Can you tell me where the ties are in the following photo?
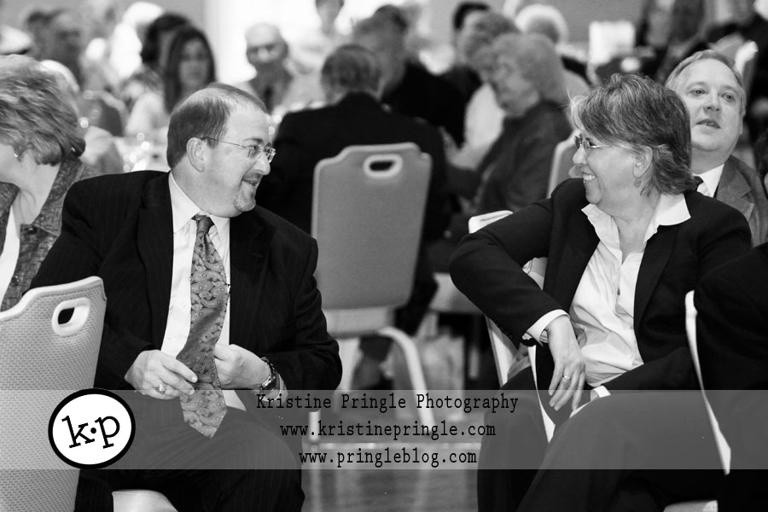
[692,176,703,190]
[176,213,228,439]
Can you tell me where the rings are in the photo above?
[563,373,572,380]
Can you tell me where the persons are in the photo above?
[1,54,103,312]
[127,17,187,113]
[662,48,767,246]
[352,33,572,389]
[440,0,574,118]
[665,2,766,59]
[310,0,410,43]
[247,25,298,110]
[259,46,447,388]
[355,20,442,122]
[453,76,768,512]
[129,24,217,142]
[0,1,84,77]
[29,85,343,511]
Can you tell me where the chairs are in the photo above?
[544,130,575,200]
[0,275,106,512]
[311,142,437,441]
[468,209,547,387]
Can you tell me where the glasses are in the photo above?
[573,133,616,153]
[201,136,277,163]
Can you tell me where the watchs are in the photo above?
[256,353,280,400]
